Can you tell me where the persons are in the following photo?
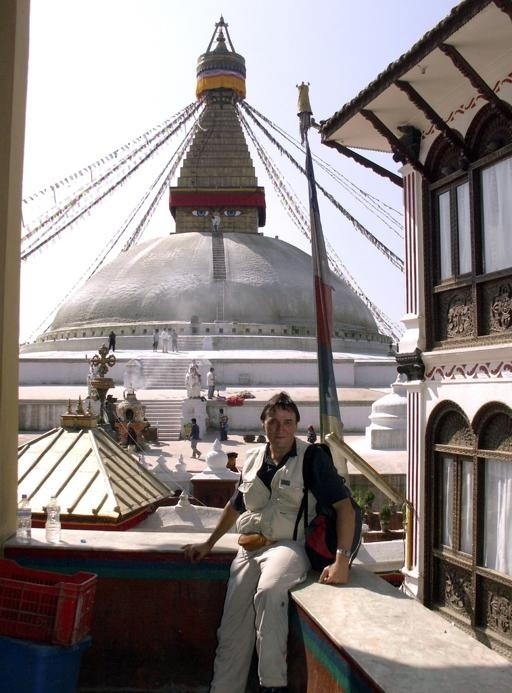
[153,327,161,352]
[108,330,116,351]
[219,407,228,440]
[189,418,201,459]
[161,327,169,352]
[180,391,357,693]
[306,424,316,443]
[207,366,216,398]
[171,329,178,351]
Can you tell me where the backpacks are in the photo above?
[293,442,363,571]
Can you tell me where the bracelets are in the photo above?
[205,539,213,548]
[336,547,350,557]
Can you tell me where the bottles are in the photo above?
[15,494,33,544]
[44,496,62,543]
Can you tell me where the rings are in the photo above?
[184,549,189,552]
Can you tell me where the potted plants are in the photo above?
[351,489,406,532]
